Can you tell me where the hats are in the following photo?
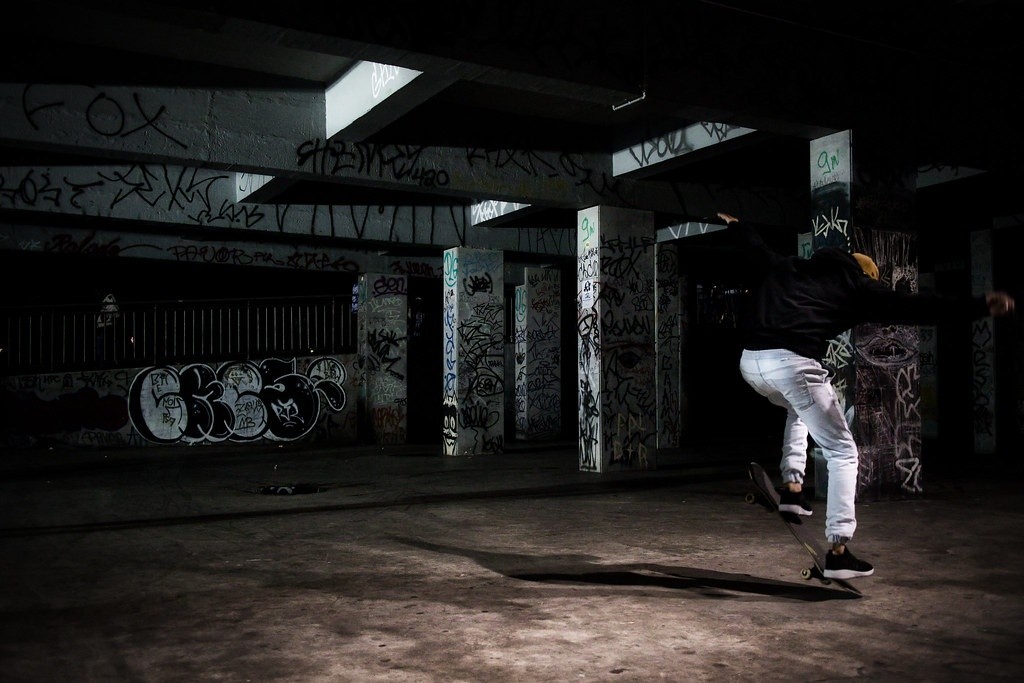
[851,252,880,281]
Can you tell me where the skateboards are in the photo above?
[744,461,864,598]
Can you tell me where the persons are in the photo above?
[717,211,1015,580]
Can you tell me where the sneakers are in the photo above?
[779,487,814,516]
[822,545,875,579]
